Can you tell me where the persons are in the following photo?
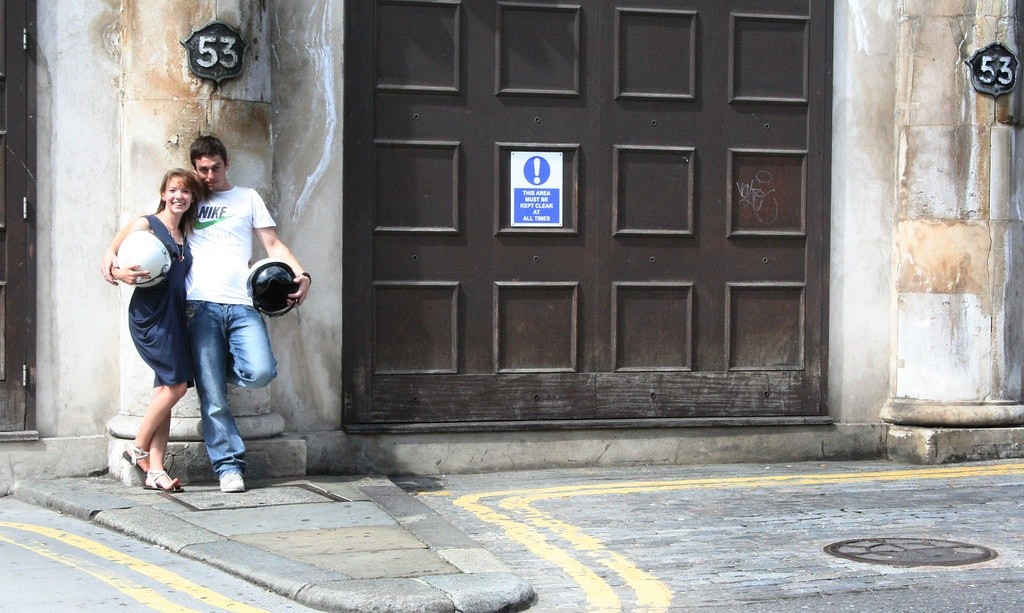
[102,132,313,495]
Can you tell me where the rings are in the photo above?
[136,276,142,282]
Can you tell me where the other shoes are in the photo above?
[221,472,244,492]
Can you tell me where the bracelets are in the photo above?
[300,272,312,284]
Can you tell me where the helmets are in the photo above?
[244,257,297,316]
[116,230,171,288]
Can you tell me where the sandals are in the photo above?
[145,472,185,492]
[123,441,149,475]
